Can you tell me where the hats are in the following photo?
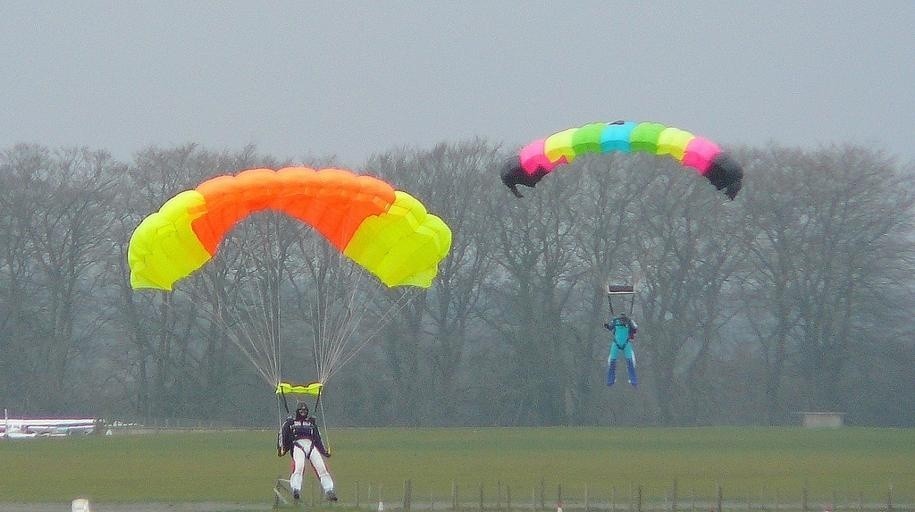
[296,401,309,412]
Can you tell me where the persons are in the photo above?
[604,312,638,387]
[278,401,337,501]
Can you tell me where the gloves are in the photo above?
[277,447,287,457]
[321,451,331,458]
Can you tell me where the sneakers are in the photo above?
[326,490,338,501]
[293,488,300,499]
